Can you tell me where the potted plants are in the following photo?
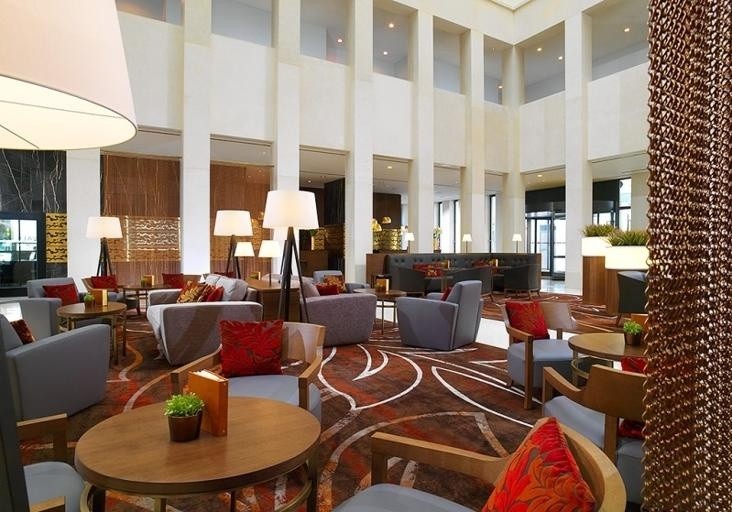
[575,220,619,257]
[604,225,654,272]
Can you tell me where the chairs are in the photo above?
[1,252,657,511]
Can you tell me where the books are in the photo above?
[376,278,390,292]
[442,259,450,269]
[189,368,228,437]
[143,275,154,285]
[492,259,498,267]
[90,288,108,307]
[631,312,649,335]
[251,271,261,280]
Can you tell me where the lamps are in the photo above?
[234,231,522,289]
[1,0,139,151]
[261,189,322,323]
[87,215,124,279]
[212,209,252,279]
